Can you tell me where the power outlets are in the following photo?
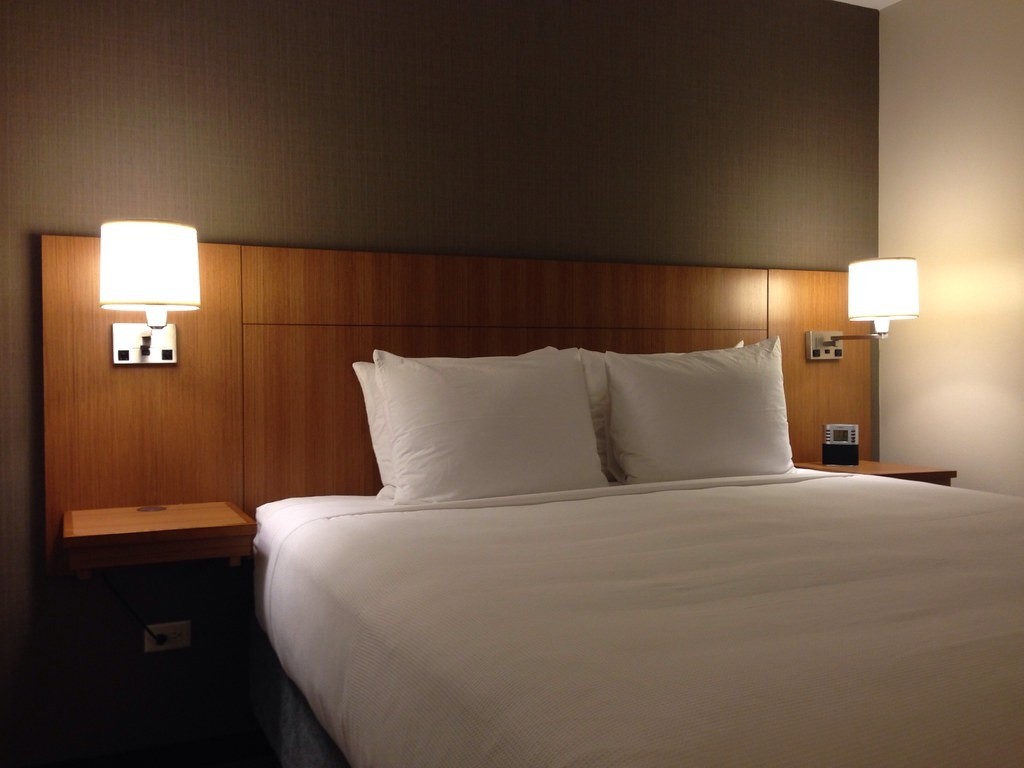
[145,620,191,653]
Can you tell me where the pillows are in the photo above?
[604,336,800,476]
[580,340,744,484]
[353,345,560,502]
[372,348,609,505]
[252,467,1024,768]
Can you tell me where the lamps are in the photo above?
[99,219,201,365]
[805,257,920,360]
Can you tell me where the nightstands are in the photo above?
[793,461,957,486]
[64,501,257,570]
